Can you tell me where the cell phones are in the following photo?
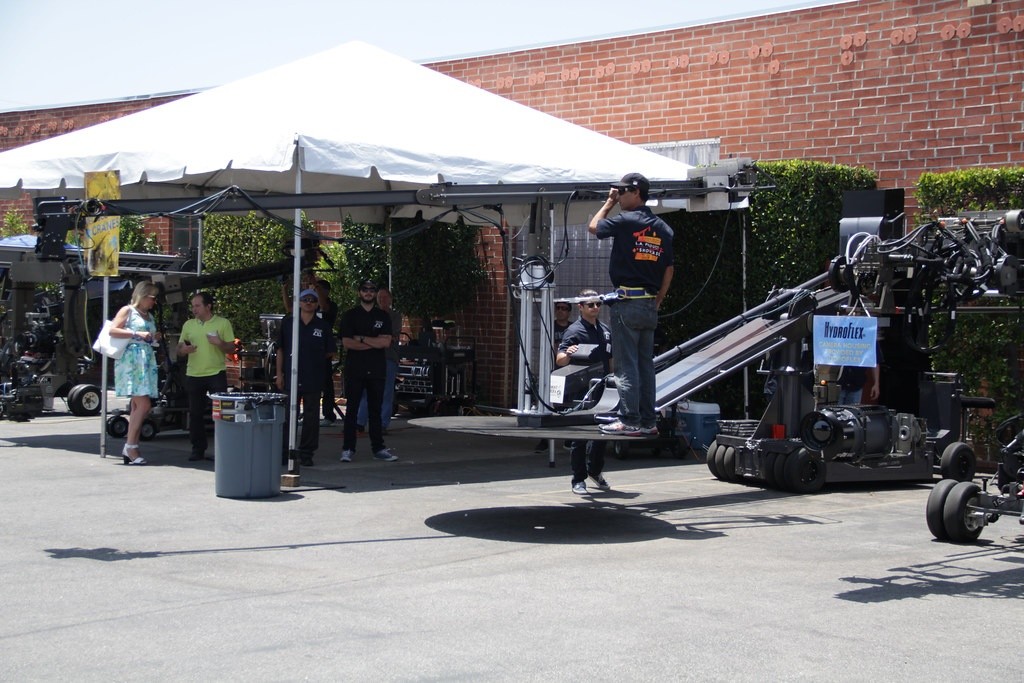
[184,340,191,346]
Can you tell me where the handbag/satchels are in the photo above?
[92,306,131,359]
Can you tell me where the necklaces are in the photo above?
[136,307,148,316]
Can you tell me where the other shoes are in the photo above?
[383,426,387,435]
[302,457,313,466]
[189,446,205,460]
[320,419,332,426]
[534,439,548,453]
[356,424,365,432]
[563,441,571,449]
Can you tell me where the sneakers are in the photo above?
[573,481,588,494]
[588,474,610,489]
[598,420,642,436]
[340,450,354,462]
[374,448,399,461]
[642,425,657,434]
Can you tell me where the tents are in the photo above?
[0,37,709,489]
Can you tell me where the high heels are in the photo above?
[122,444,147,464]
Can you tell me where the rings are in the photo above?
[160,336,162,339]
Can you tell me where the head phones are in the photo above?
[527,255,555,284]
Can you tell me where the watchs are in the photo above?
[360,335,365,343]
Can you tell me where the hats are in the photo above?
[300,289,318,298]
[610,173,650,192]
[359,279,376,290]
[316,280,331,289]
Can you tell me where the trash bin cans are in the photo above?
[210,391,288,499]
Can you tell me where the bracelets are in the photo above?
[132,330,136,339]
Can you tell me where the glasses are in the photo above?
[555,306,569,311]
[301,298,316,303]
[582,302,601,308]
[618,187,636,195]
[147,295,159,302]
[362,286,376,292]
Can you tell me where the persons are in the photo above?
[298,269,337,426]
[339,280,399,461]
[836,338,886,409]
[356,287,402,435]
[588,172,675,436]
[274,290,339,467]
[533,302,575,453]
[556,289,614,497]
[109,280,162,465]
[175,292,235,461]
